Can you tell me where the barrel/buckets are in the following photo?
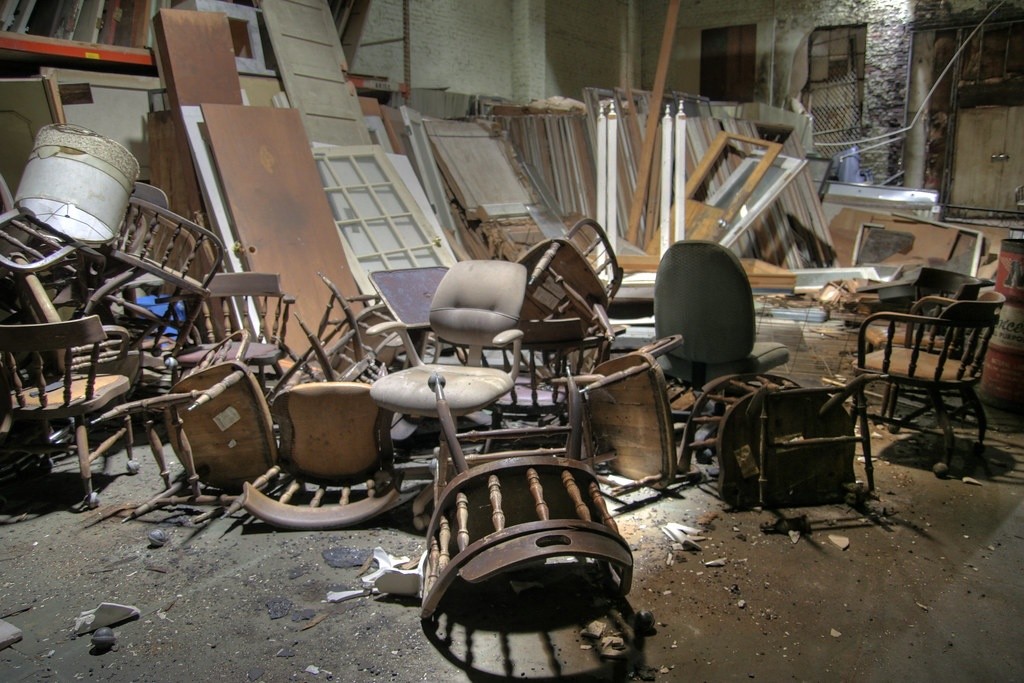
[979,238,1023,414]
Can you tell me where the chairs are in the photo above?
[0,173,1008,624]
